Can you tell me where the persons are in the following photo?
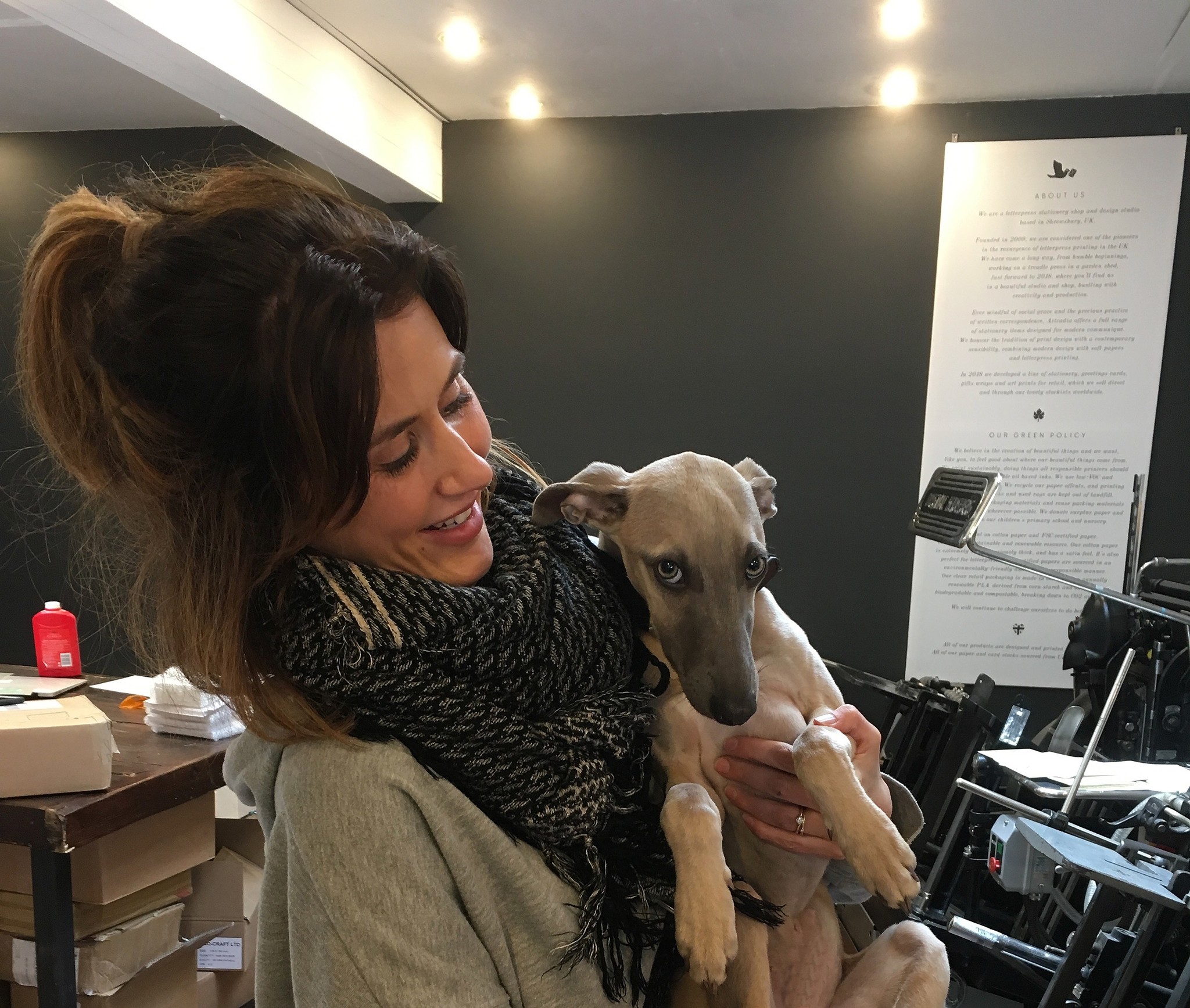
[20,163,926,1008]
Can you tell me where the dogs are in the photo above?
[530,449,951,1008]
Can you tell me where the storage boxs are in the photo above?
[0,695,265,1008]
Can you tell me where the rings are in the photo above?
[795,805,806,835]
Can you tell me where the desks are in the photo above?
[0,664,242,1008]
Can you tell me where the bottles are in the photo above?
[32,601,81,677]
